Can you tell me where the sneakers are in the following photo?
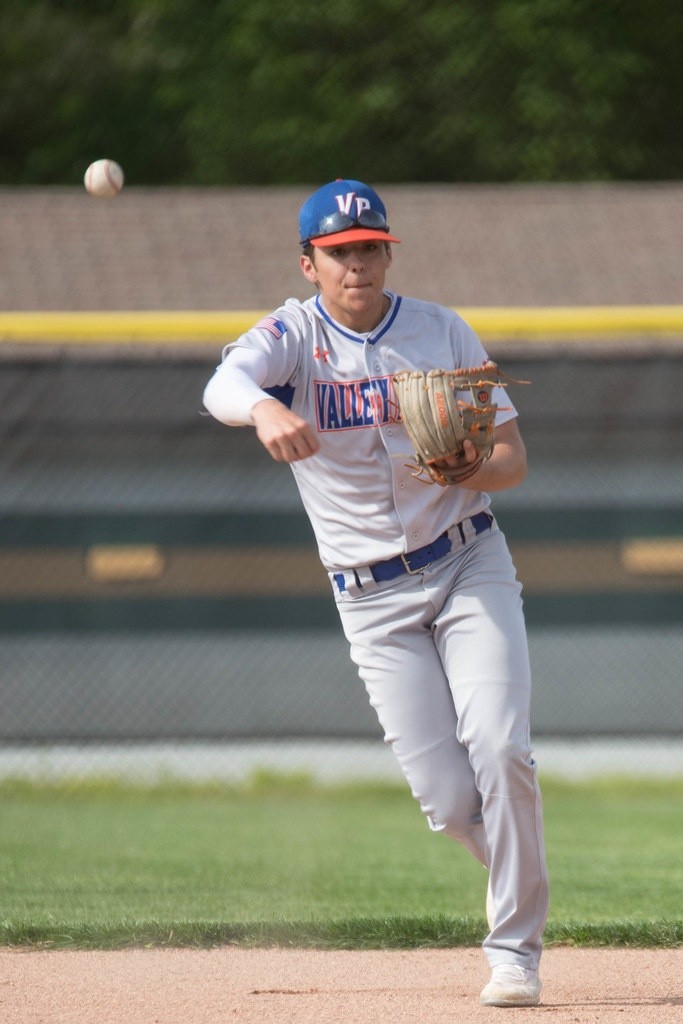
[479,963,543,1008]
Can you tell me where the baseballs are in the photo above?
[82,159,124,196]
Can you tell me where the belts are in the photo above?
[334,513,492,591]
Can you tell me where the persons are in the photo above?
[202,179,549,1006]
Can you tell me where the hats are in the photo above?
[298,177,402,248]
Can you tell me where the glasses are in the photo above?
[300,210,390,244]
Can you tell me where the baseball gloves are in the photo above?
[390,362,531,484]
